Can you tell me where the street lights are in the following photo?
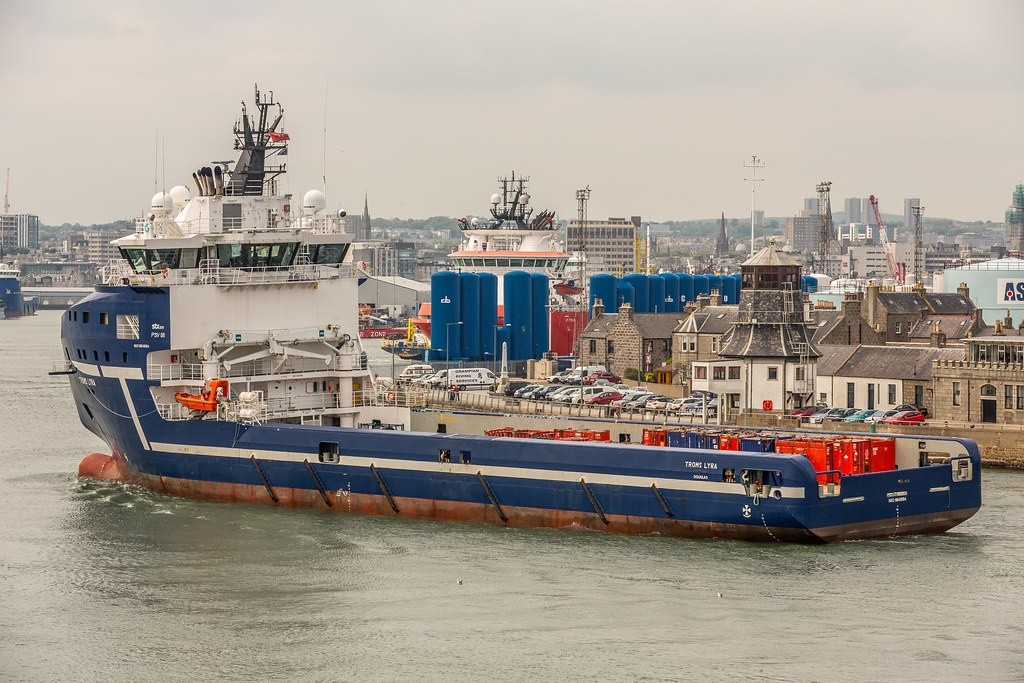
[446,321,464,392]
[492,324,512,394]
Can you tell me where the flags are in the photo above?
[268,133,290,142]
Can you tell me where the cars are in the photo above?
[547,372,569,384]
[892,404,928,417]
[410,370,435,386]
[810,408,843,424]
[515,372,735,417]
[885,411,925,427]
[561,373,573,384]
[864,410,899,424]
[843,410,878,422]
[826,408,862,422]
[787,405,830,423]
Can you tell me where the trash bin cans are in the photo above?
[603,407,614,418]
[447,389,455,400]
[870,424,876,434]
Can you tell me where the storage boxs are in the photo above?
[482,428,898,486]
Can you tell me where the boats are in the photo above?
[0,263,40,318]
[48,84,982,543]
[408,170,589,357]
[381,318,431,362]
[358,306,413,339]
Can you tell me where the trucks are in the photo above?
[568,365,606,386]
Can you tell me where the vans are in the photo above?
[399,364,433,381]
[430,370,448,388]
[504,380,531,397]
[449,368,499,391]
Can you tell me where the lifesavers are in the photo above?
[386,392,395,402]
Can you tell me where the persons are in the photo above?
[454,385,459,401]
[610,400,615,418]
[615,403,621,419]
